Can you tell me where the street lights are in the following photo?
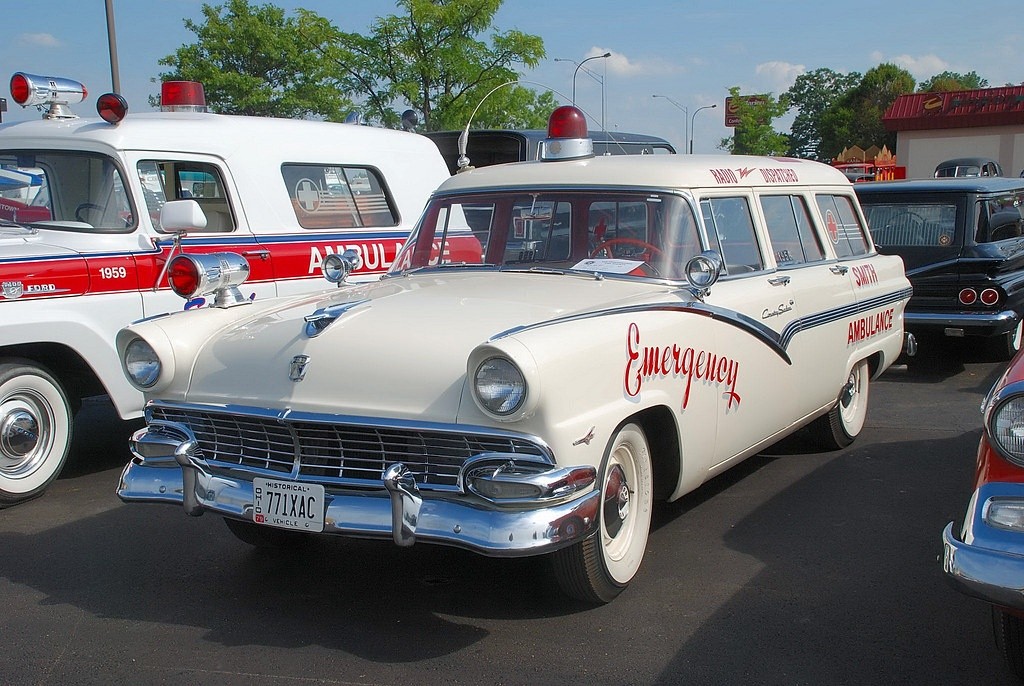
[553,58,606,131]
[652,93,689,155]
[572,53,612,109]
[690,104,716,155]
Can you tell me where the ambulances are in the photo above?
[1,70,486,506]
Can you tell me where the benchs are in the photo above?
[115,210,232,234]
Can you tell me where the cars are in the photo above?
[113,104,917,606]
[940,348,1024,682]
[395,128,1024,361]
